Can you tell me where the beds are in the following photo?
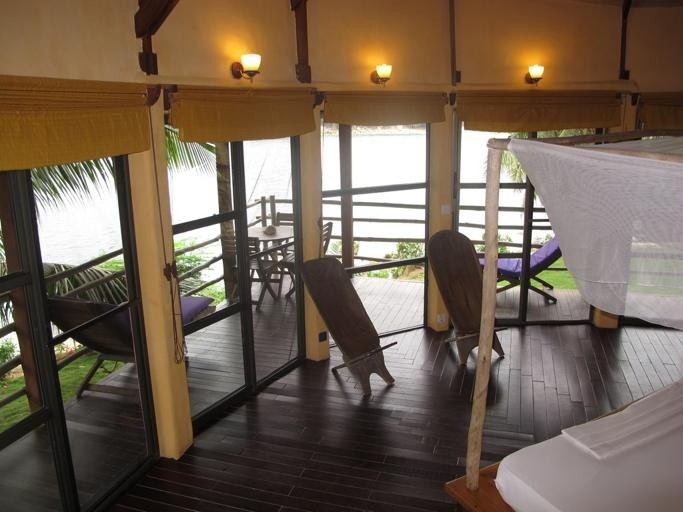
[440,379,683,512]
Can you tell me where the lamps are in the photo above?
[525,64,544,87]
[370,64,393,88]
[231,54,261,84]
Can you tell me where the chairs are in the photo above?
[47,296,216,404]
[478,235,562,302]
[229,212,332,310]
